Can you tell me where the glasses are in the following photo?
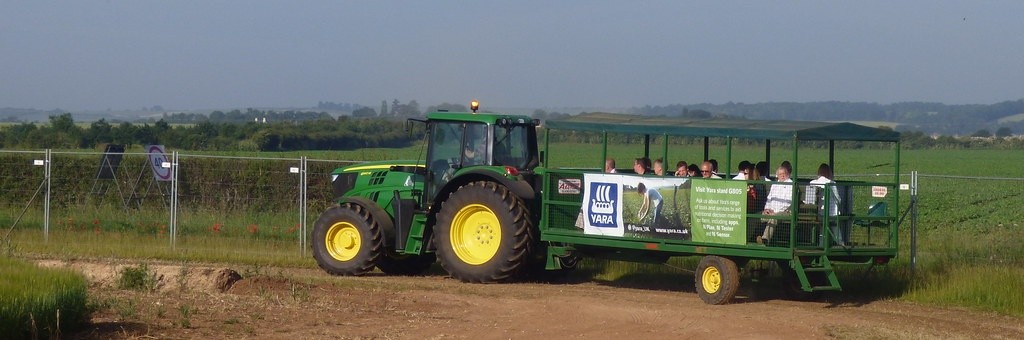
[700,170,711,172]
[744,172,749,175]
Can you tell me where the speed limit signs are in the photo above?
[145,146,174,184]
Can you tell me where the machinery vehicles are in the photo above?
[310,98,919,306]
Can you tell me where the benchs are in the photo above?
[748,186,850,224]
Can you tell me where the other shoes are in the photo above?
[757,236,764,245]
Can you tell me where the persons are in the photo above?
[464,139,475,161]
[781,161,792,174]
[809,162,845,247]
[732,160,775,213]
[633,157,722,180]
[638,183,663,223]
[756,166,799,247]
[606,158,617,173]
